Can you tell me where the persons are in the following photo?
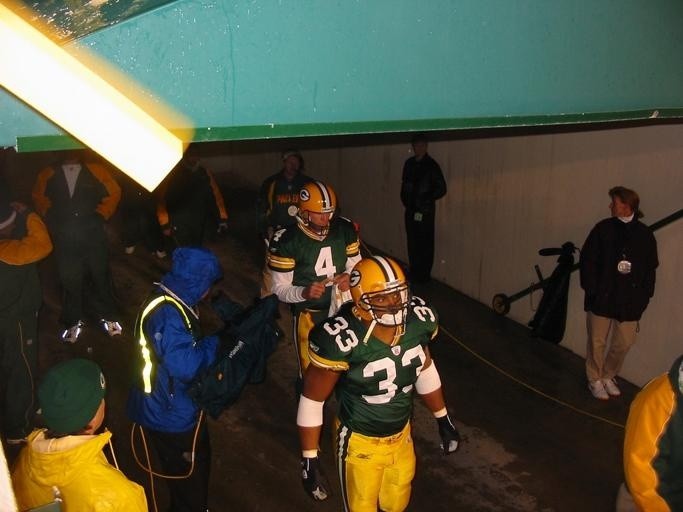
[32,151,123,346]
[296,256,459,512]
[125,247,229,512]
[0,200,54,445]
[615,355,682,512]
[579,186,659,399]
[263,180,362,379]
[262,148,303,247]
[123,196,167,260]
[12,358,148,512]
[399,133,448,285]
[156,146,228,248]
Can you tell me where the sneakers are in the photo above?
[98,317,123,337]
[602,377,620,395]
[588,380,609,400]
[124,243,135,255]
[61,319,85,344]
[154,247,167,261]
[7,425,45,445]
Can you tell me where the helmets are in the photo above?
[299,180,336,237]
[348,255,409,327]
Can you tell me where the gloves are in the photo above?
[436,411,460,454]
[217,222,230,234]
[300,456,327,502]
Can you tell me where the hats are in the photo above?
[0,201,16,231]
[37,357,108,432]
